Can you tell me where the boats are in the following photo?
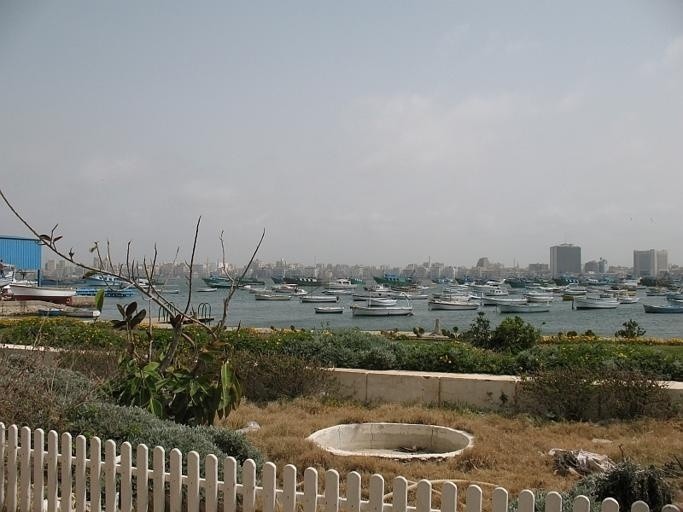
[571,295,621,309]
[495,302,553,313]
[195,287,218,293]
[9,275,179,318]
[313,306,345,314]
[350,291,415,316]
[643,303,683,314]
[201,271,237,288]
[427,294,481,311]
[236,272,683,306]
